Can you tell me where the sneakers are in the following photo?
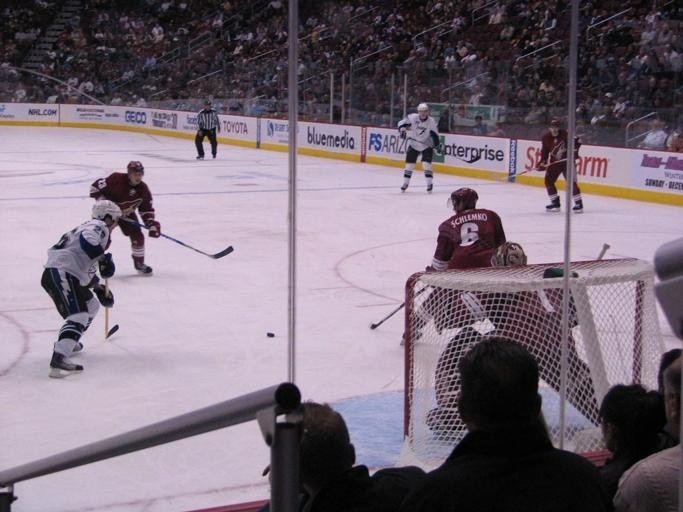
[401,183,408,190]
[50,351,83,371]
[131,255,153,274]
[572,199,583,210]
[403,329,422,339]
[72,343,83,352]
[196,155,204,159]
[426,184,433,191]
[546,196,560,210]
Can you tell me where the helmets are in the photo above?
[449,188,479,202]
[92,200,123,222]
[549,120,561,127]
[127,162,144,171]
[417,103,428,110]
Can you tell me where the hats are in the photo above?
[491,242,527,268]
[604,93,613,97]
[203,99,211,105]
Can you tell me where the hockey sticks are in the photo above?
[370,286,427,332]
[104,277,120,340]
[123,216,234,259]
[407,136,481,164]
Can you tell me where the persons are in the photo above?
[657,348,682,396]
[473,104,567,137]
[89,160,160,273]
[612,358,683,512]
[595,382,665,499]
[40,200,123,371]
[194,101,220,159]
[297,400,426,512]
[398,0,569,103]
[425,337,598,512]
[537,119,584,213]
[577,1,683,152]
[453,241,609,425]
[298,0,397,127]
[397,103,440,191]
[401,187,505,340]
[0,0,221,105]
[221,1,288,118]
